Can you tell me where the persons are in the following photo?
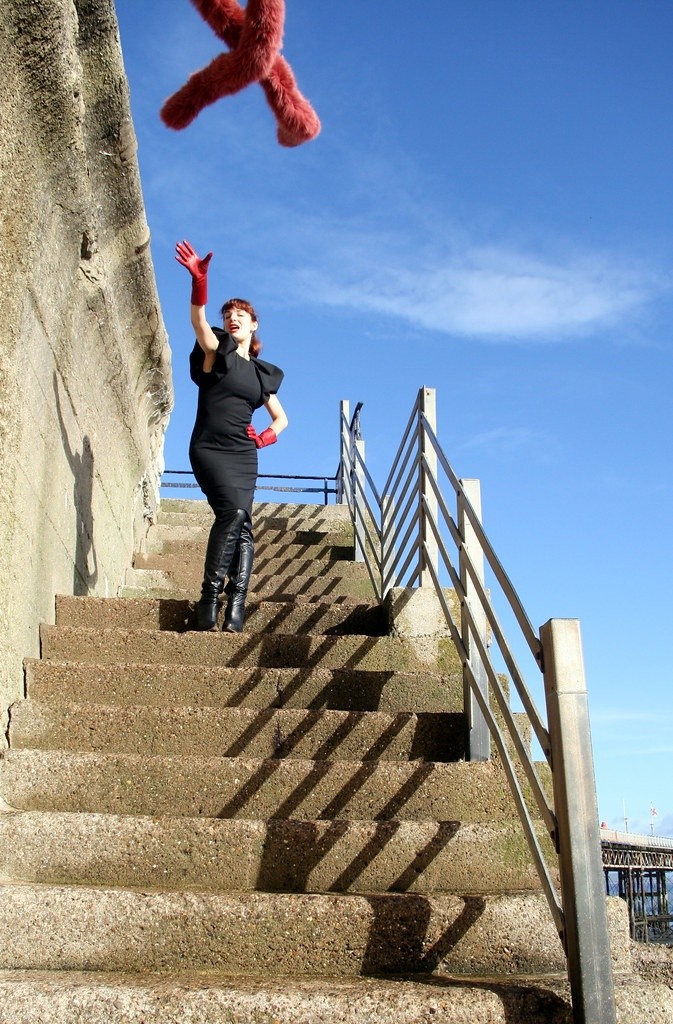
[175,240,289,633]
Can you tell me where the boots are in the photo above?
[195,509,247,632]
[223,526,255,633]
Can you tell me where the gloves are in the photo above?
[175,240,213,306]
[247,424,278,449]
